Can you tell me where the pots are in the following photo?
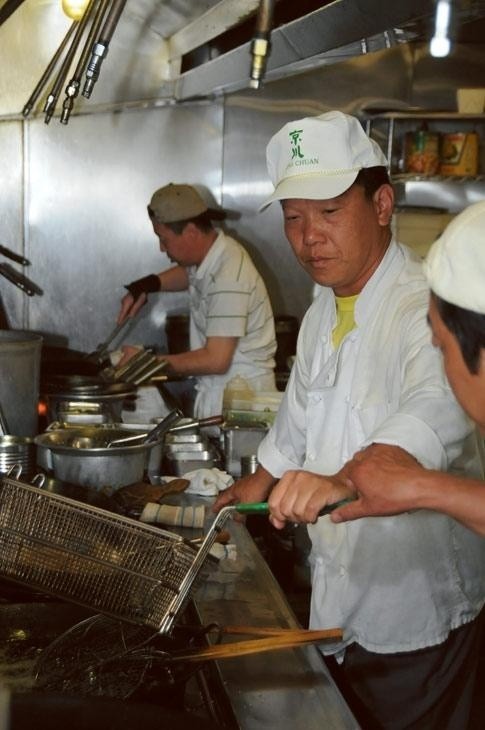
[40,374,135,424]
[32,419,164,499]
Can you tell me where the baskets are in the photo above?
[1,474,219,632]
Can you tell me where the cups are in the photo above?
[456,86,485,116]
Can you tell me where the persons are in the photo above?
[210,109,485,730]
[119,181,278,477]
[329,198,485,544]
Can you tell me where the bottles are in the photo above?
[239,455,259,478]
[223,374,252,410]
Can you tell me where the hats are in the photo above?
[422,200,484,314]
[257,109,389,214]
[148,182,227,224]
